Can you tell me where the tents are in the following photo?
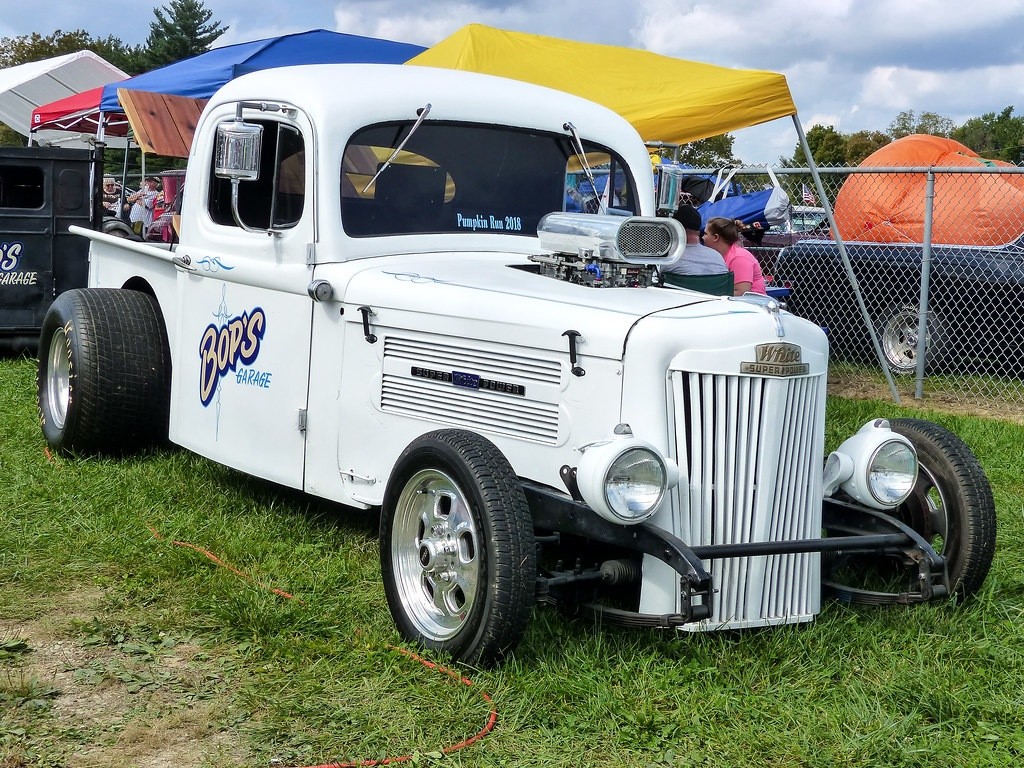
[0,49,133,221]
[406,27,902,402]
[29,29,430,249]
[829,133,1023,252]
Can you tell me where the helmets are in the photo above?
[103,173,116,193]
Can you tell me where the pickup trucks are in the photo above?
[36,65,998,676]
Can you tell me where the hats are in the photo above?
[672,205,702,231]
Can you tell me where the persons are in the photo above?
[701,217,767,297]
[102,174,130,225]
[668,205,729,274]
[128,176,164,239]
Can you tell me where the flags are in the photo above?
[802,184,817,207]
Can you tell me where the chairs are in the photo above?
[374,161,448,233]
